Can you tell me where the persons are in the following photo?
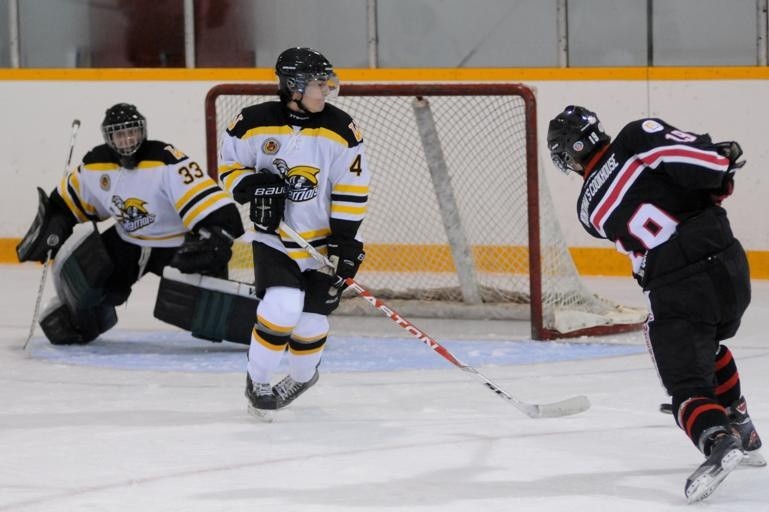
[547,105,768,503]
[220,46,370,411]
[120,0,229,69]
[14,102,259,346]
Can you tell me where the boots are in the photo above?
[684,395,763,502]
[244,347,322,410]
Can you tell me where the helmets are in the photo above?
[275,46,340,107]
[547,105,612,176]
[101,102,147,158]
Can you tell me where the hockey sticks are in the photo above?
[22,119,80,350]
[280,221,590,417]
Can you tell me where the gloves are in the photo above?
[249,173,290,231]
[325,234,366,294]
